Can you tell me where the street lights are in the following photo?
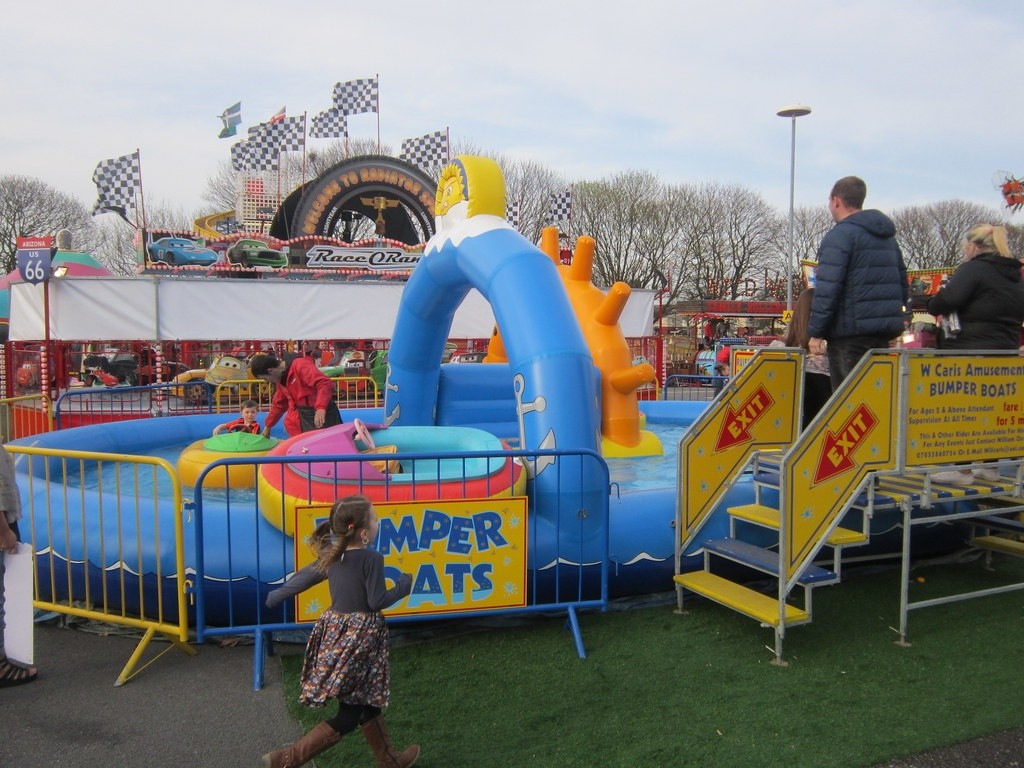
[777,105,810,319]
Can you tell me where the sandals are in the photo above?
[0,655,38,687]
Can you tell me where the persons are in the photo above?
[309,341,335,367]
[693,319,716,364]
[0,441,38,690]
[213,399,260,436]
[808,176,910,396]
[261,493,420,768]
[251,352,343,438]
[926,224,1024,349]
[712,364,729,402]
[717,319,751,339]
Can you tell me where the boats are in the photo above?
[177,426,288,487]
[258,418,512,531]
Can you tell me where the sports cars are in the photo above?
[146,237,219,265]
[228,239,288,269]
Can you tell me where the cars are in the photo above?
[172,368,273,404]
[109,348,190,385]
[317,349,388,398]
[203,354,250,393]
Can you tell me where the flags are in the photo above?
[506,201,521,230]
[399,129,448,171]
[215,102,305,171]
[309,76,379,138]
[543,185,573,223]
[92,152,144,230]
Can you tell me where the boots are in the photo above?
[361,713,420,768]
[262,718,341,768]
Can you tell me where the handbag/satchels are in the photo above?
[296,403,343,432]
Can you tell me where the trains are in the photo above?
[16,351,58,391]
